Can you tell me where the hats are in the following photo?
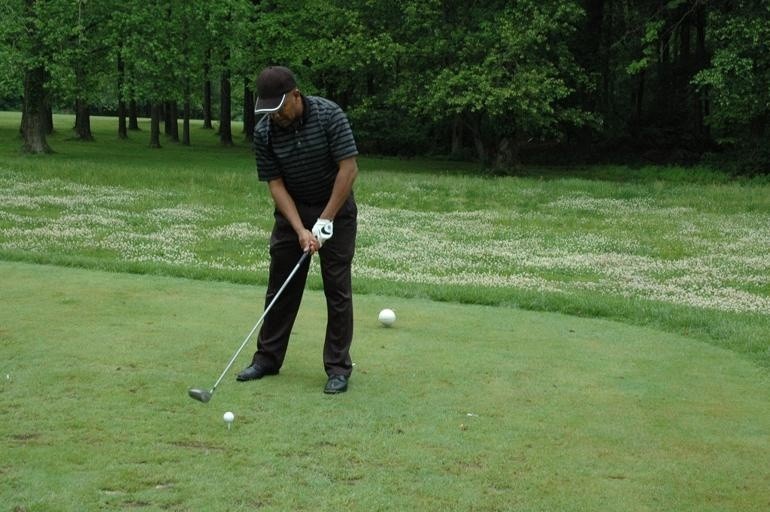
[254,66,296,115]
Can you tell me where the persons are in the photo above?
[233,67,362,394]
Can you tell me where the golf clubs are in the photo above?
[188,250,310,404]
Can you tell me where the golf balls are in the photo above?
[379,309,395,324]
[224,412,234,422]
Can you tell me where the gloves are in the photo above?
[311,218,334,247]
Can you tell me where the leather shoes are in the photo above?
[323,374,348,394]
[237,364,279,381]
